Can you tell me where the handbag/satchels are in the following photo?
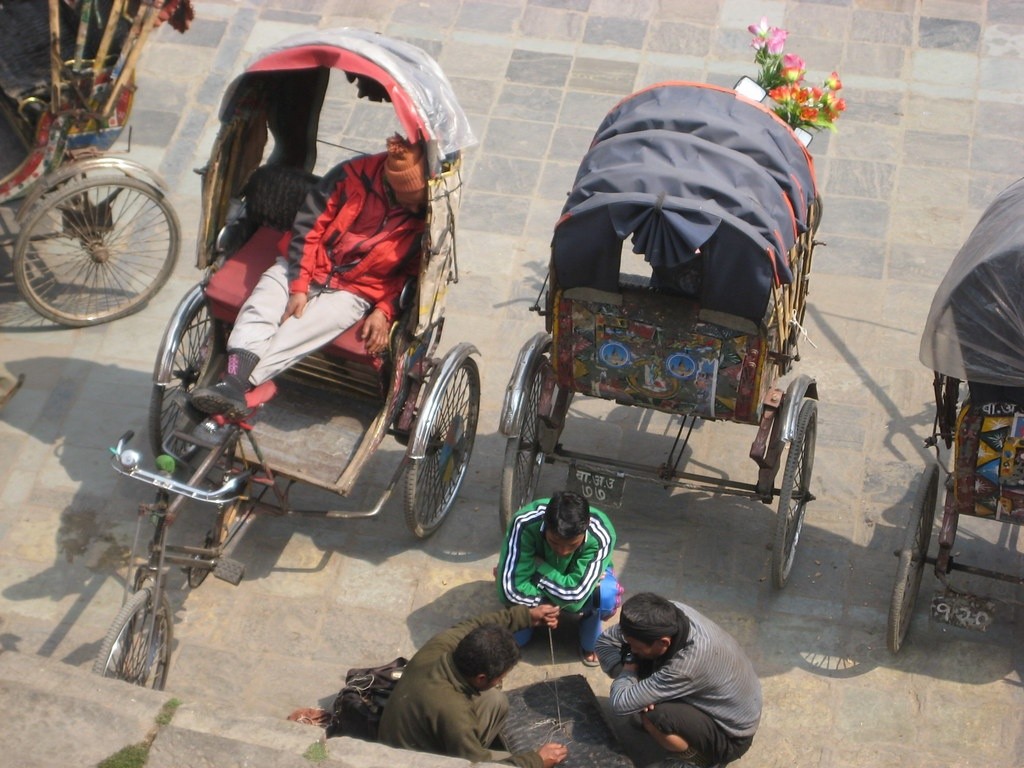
[289,708,332,729]
[329,656,414,743]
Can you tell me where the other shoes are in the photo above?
[628,713,644,730]
[648,752,721,768]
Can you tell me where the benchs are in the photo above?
[200,165,429,361]
[1,0,129,124]
[550,287,761,425]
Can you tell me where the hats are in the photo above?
[384,133,430,194]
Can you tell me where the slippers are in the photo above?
[579,641,601,666]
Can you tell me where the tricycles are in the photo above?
[498,77,819,587]
[89,26,485,700]
[883,181,1024,654]
[0,0,198,326]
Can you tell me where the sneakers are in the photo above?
[191,380,250,419]
[194,415,232,445]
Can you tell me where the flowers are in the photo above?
[748,16,846,137]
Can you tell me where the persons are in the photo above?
[378,604,567,768]
[595,592,763,768]
[496,492,616,667]
[172,133,428,435]
